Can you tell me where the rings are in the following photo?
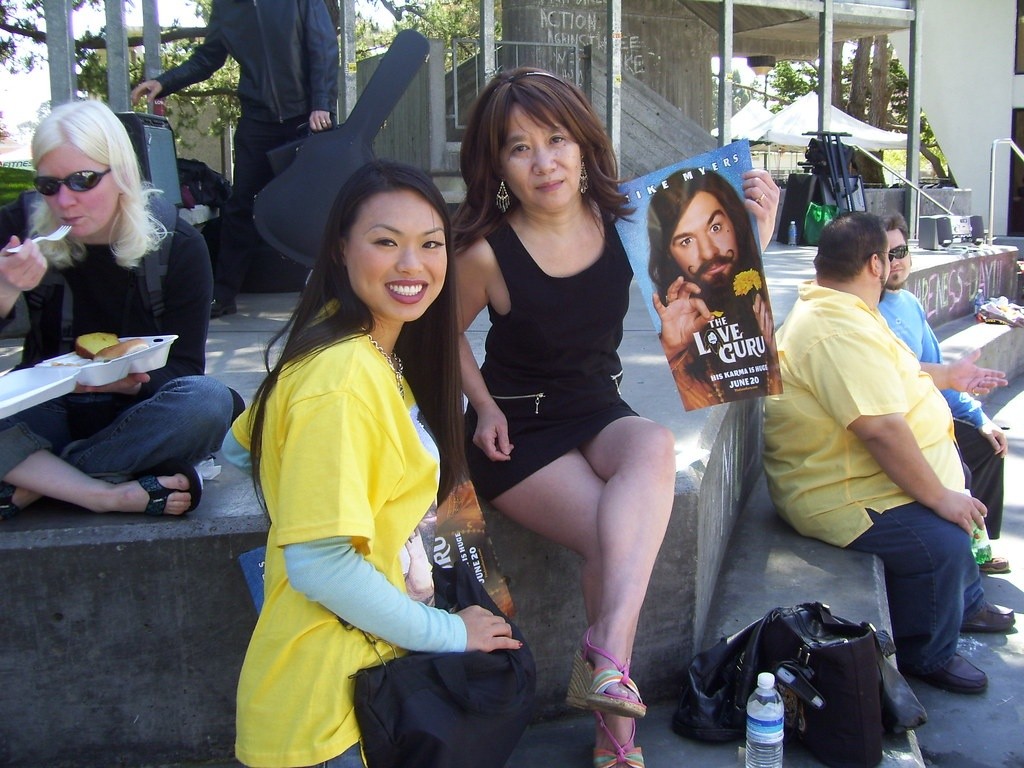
[755,193,765,203]
[973,389,979,397]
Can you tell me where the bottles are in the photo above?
[963,489,992,565]
[746,673,784,768]
[787,221,797,246]
[975,289,984,315]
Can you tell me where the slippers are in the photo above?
[101,462,204,516]
[0,482,20,522]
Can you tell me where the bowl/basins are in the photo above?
[35,335,179,385]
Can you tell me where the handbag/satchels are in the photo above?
[806,138,853,176]
[802,202,841,247]
[346,560,537,768]
[758,601,883,768]
[261,122,343,178]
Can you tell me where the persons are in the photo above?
[446,64,782,768]
[403,524,435,604]
[761,210,1015,693]
[877,211,1010,574]
[646,167,783,412]
[221,157,524,768]
[131,0,339,319]
[0,98,246,516]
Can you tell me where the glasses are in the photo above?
[866,250,895,262]
[33,168,111,195]
[894,245,909,259]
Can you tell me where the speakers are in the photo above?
[112,113,184,206]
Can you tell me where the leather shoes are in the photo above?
[961,600,1015,632]
[210,298,236,316]
[898,652,988,692]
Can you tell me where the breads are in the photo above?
[76,332,150,362]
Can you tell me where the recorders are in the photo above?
[918,214,984,252]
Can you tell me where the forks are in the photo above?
[6,225,72,252]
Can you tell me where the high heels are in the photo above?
[591,707,645,768]
[565,625,648,718]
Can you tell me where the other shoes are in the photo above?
[978,557,1009,573]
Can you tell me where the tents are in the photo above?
[711,98,776,147]
[732,91,907,150]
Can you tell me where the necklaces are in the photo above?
[361,327,405,401]
[703,347,770,403]
